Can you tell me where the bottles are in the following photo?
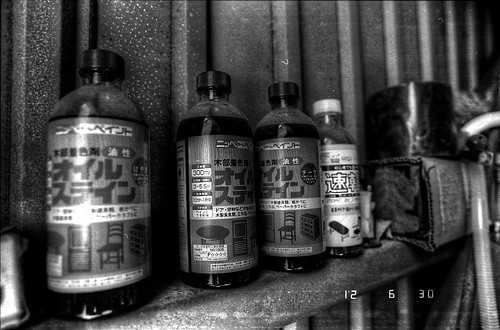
[311,98,364,259]
[176,70,259,289]
[255,82,327,274]
[43,50,152,319]
